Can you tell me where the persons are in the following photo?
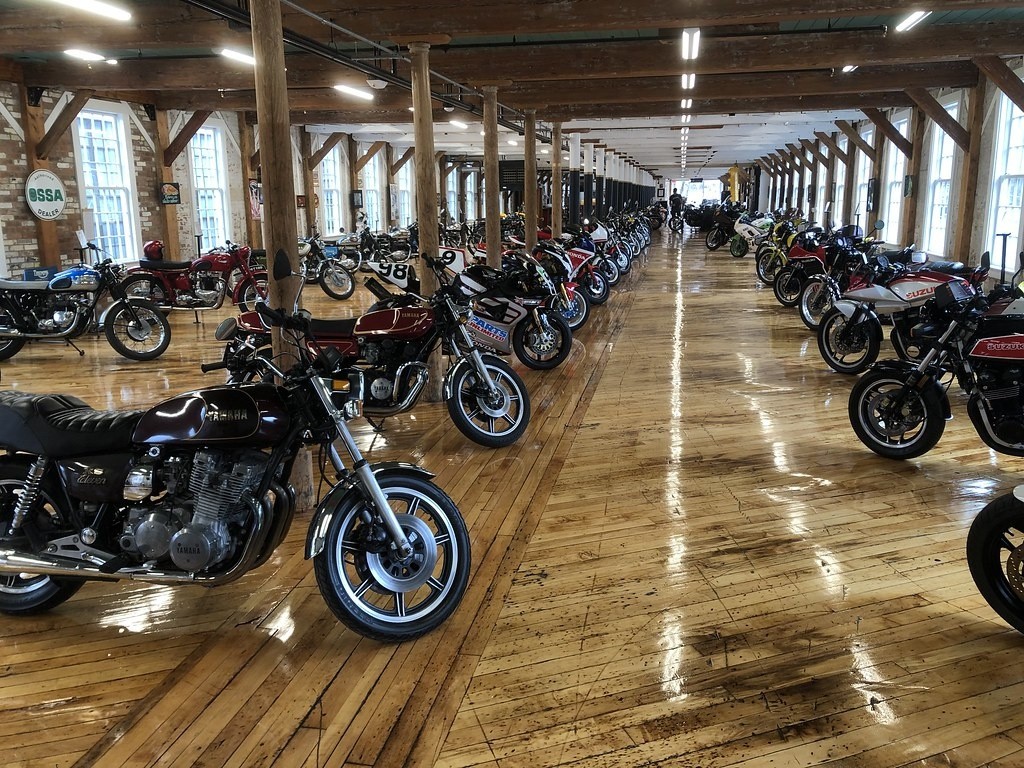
[669,188,685,234]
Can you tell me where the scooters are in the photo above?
[967,485,1024,636]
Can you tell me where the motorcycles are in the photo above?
[0,244,471,639]
[220,199,667,447]
[0,242,171,364]
[117,239,269,328]
[668,195,992,374]
[848,251,1024,460]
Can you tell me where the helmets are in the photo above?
[143,241,165,259]
